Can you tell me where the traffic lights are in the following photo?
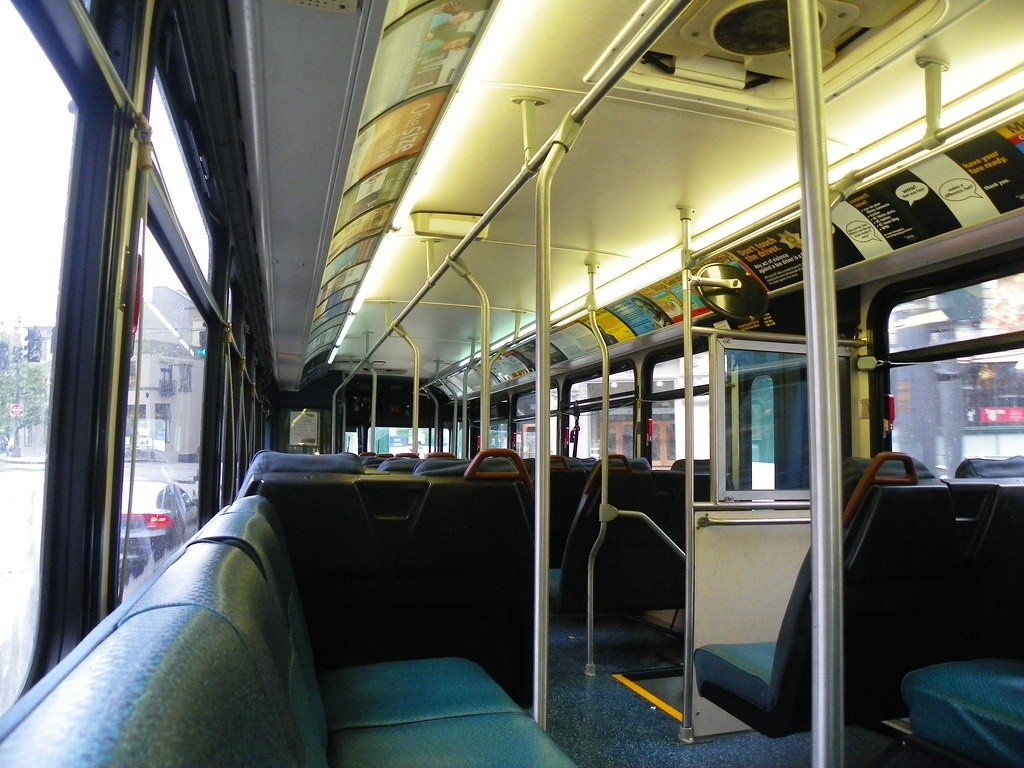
[23,328,42,362]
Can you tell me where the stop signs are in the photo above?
[10,404,24,417]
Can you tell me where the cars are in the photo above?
[120,446,199,587]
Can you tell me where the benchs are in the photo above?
[0,452,1024,768]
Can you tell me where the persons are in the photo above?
[589,322,619,346]
[421,8,475,60]
[631,297,671,330]
